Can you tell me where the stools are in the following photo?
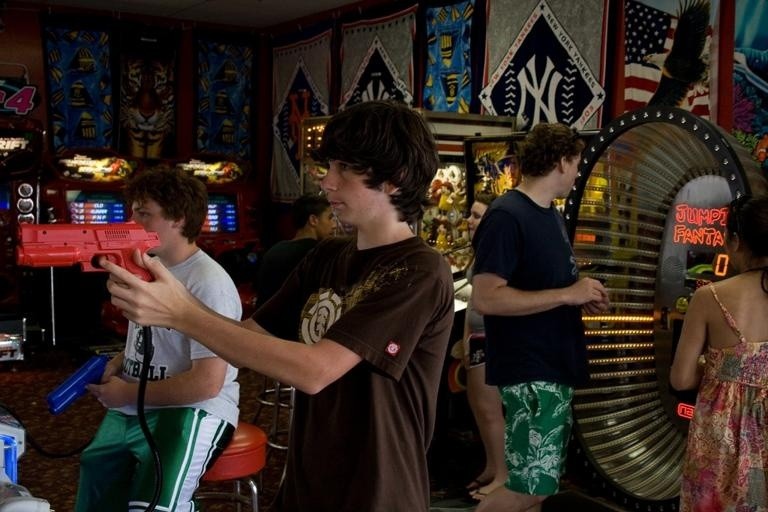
[190,420,269,512]
[243,374,300,512]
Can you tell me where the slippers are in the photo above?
[463,471,492,504]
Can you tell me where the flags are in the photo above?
[620,0,713,124]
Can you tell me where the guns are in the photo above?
[16,221,160,282]
[47,355,111,414]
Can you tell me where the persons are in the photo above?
[497,142,524,188]
[667,195,767,511]
[70,164,243,511]
[253,193,338,313]
[473,121,611,511]
[99,98,456,511]
[460,191,511,501]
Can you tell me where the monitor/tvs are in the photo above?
[196,192,240,233]
[66,189,127,225]
